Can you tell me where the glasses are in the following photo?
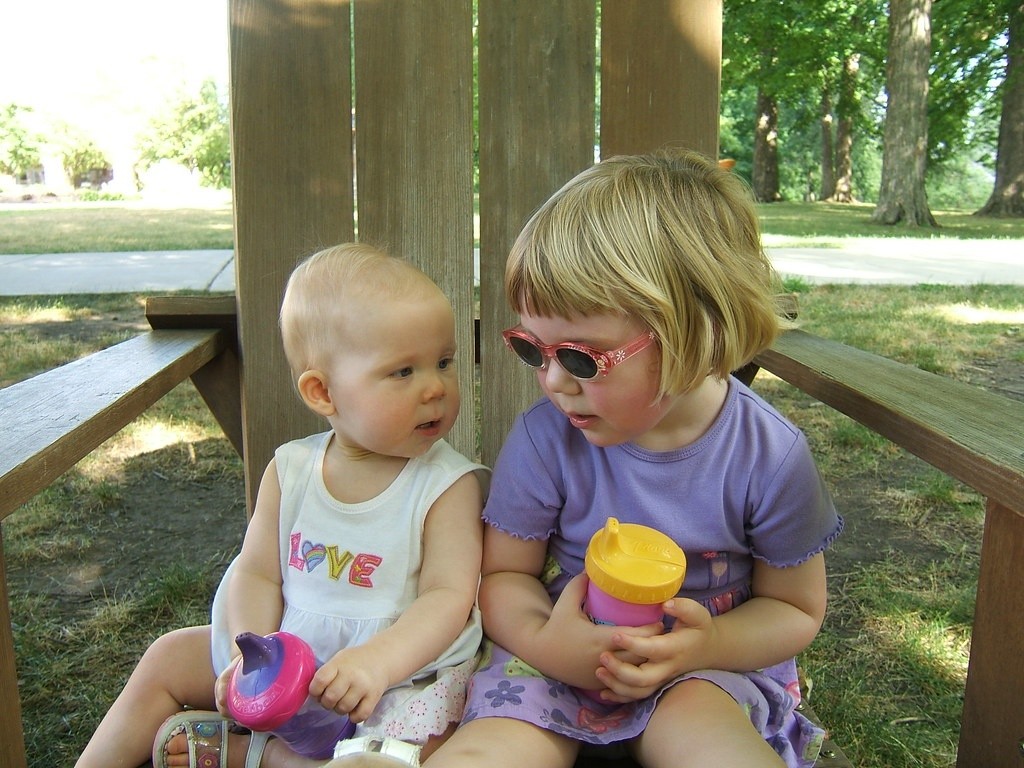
[500,323,656,383]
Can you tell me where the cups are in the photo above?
[584,514,687,706]
[227,632,356,760]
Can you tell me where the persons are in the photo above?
[74,242,493,768]
[420,150,843,768]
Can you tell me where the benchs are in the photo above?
[1,0,1024,768]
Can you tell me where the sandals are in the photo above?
[151,710,276,768]
